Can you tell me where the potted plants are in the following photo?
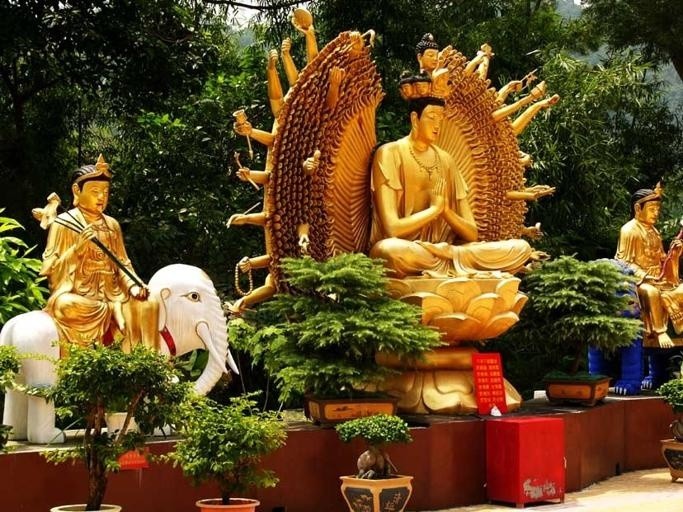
[227,251,449,424]
[522,253,647,408]
[654,378,683,482]
[335,412,414,512]
[145,390,288,512]
[24,328,196,511]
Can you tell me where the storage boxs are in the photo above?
[485,417,565,508]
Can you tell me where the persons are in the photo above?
[220,6,344,318]
[416,33,438,75]
[415,71,429,97]
[462,41,559,244]
[370,99,530,273]
[612,183,683,349]
[398,72,415,98]
[40,164,150,340]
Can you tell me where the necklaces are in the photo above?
[407,139,442,182]
